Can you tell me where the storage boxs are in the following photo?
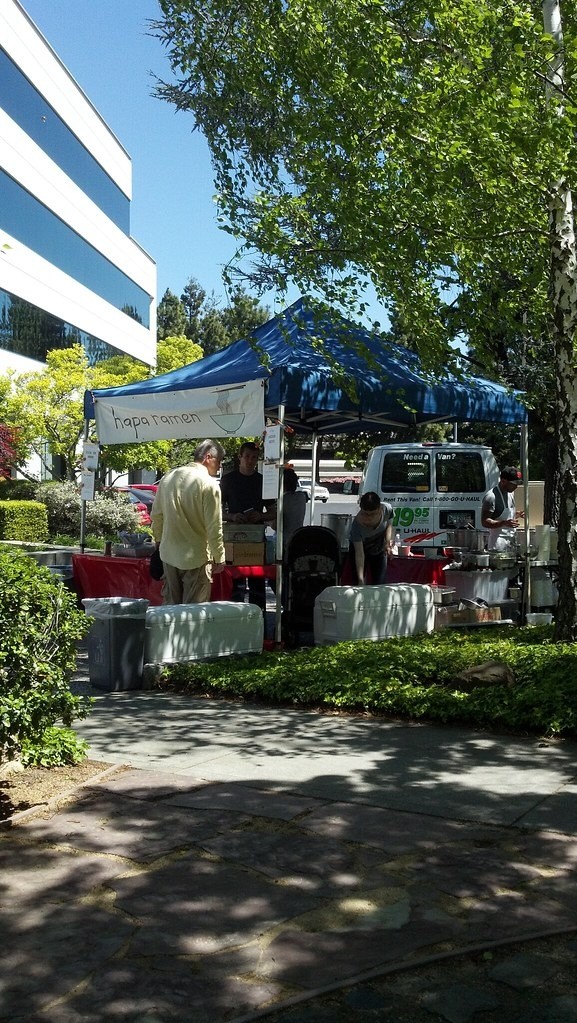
[222,541,265,566]
[433,606,502,630]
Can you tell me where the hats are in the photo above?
[501,468,523,485]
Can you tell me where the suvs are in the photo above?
[296,478,329,504]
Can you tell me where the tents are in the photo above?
[78,294,530,645]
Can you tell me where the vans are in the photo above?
[342,442,500,551]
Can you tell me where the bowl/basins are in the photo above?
[442,546,469,557]
[398,546,410,556]
[535,524,551,561]
[118,533,147,545]
[424,548,438,559]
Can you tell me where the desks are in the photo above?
[71,553,277,602]
[339,550,454,589]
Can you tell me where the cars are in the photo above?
[98,484,158,526]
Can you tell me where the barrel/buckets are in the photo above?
[525,612,553,628]
[321,514,352,552]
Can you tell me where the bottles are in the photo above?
[395,534,401,547]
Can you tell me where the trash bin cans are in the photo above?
[79,596,150,692]
[46,564,75,594]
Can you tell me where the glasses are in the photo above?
[361,509,380,517]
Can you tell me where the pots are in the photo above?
[445,525,488,551]
[492,550,516,568]
[432,585,456,607]
[460,550,490,568]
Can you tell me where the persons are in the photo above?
[267,468,306,614]
[219,442,277,640]
[481,466,525,600]
[149,438,226,606]
[340,491,393,587]
